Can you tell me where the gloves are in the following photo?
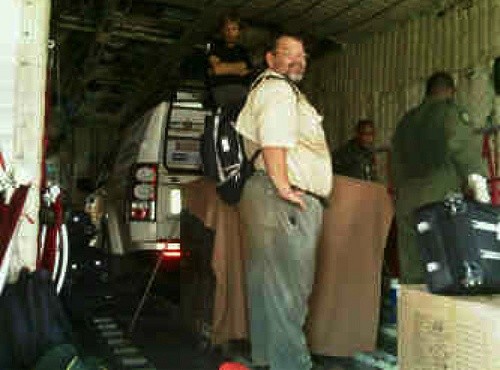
[469,173,491,203]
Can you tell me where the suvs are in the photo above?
[95,98,208,286]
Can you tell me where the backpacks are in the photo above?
[205,76,300,204]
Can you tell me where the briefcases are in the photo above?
[414,190,498,296]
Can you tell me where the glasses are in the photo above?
[271,48,310,59]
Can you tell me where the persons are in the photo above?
[205,15,260,122]
[234,31,334,370]
[330,120,379,181]
[388,72,491,286]
